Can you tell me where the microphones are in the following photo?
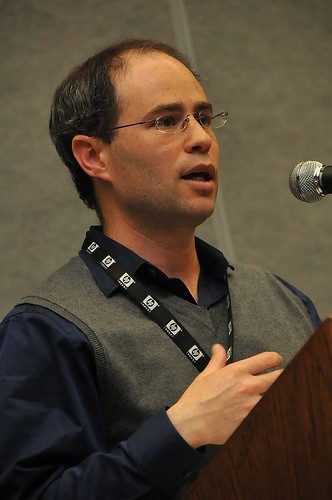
[288,160,332,202]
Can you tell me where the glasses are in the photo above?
[90,107,229,134]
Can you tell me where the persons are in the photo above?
[2,38,324,500]
